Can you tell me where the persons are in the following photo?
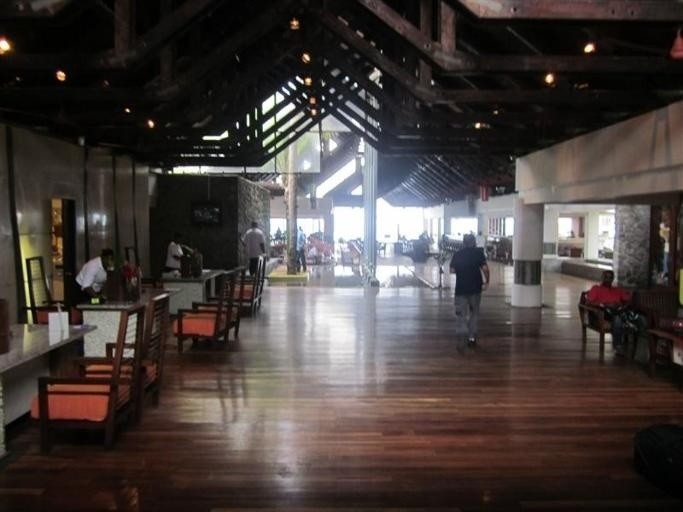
[587,269,634,361]
[238,221,267,277]
[456,230,488,260]
[296,226,338,272]
[566,230,576,239]
[278,238,283,254]
[411,231,449,272]
[338,237,350,253]
[165,234,187,273]
[70,247,115,314]
[382,234,394,253]
[505,235,513,266]
[450,234,490,345]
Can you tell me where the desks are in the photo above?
[0,319,98,455]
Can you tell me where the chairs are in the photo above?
[71,292,171,421]
[576,278,682,379]
[268,231,518,282]
[17,246,270,359]
[30,303,145,455]
